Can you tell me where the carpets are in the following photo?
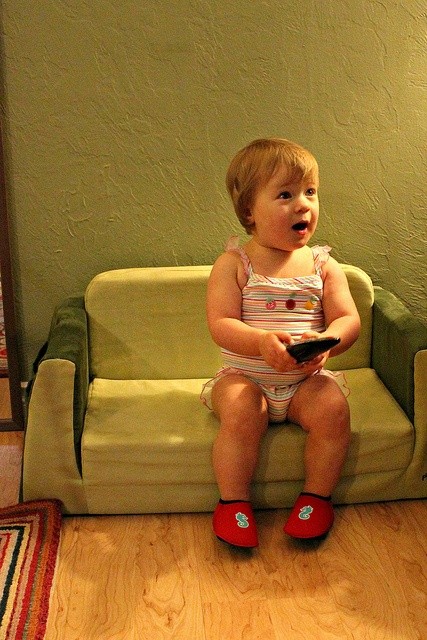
[0,497,63,640]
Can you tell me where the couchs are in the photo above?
[19,262,427,514]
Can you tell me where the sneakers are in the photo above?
[284,491,336,542]
[212,499,259,550]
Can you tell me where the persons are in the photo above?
[199,137,362,548]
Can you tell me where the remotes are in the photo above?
[282,337,343,362]
